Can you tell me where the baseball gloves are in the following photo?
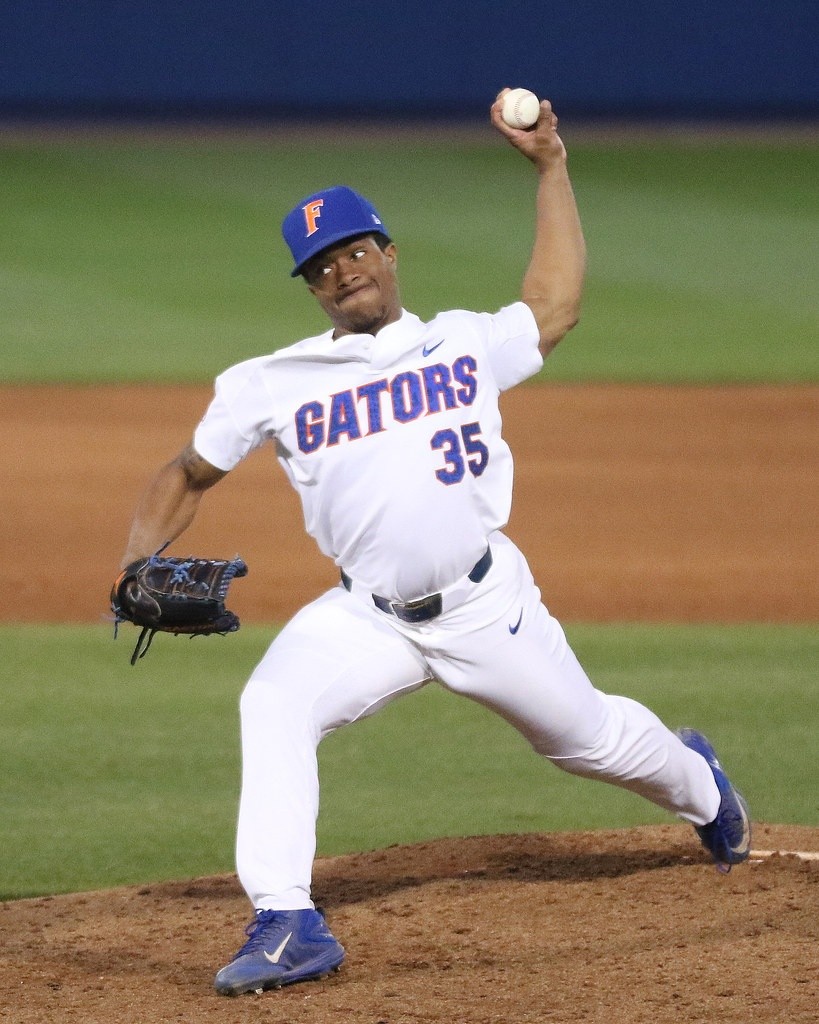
[108,542,248,667]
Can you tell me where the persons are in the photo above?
[110,85,748,996]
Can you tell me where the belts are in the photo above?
[340,546,492,622]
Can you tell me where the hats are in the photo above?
[282,186,390,278]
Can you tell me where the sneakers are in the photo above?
[673,726,751,864]
[214,908,345,994]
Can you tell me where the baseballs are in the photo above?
[501,88,539,129]
